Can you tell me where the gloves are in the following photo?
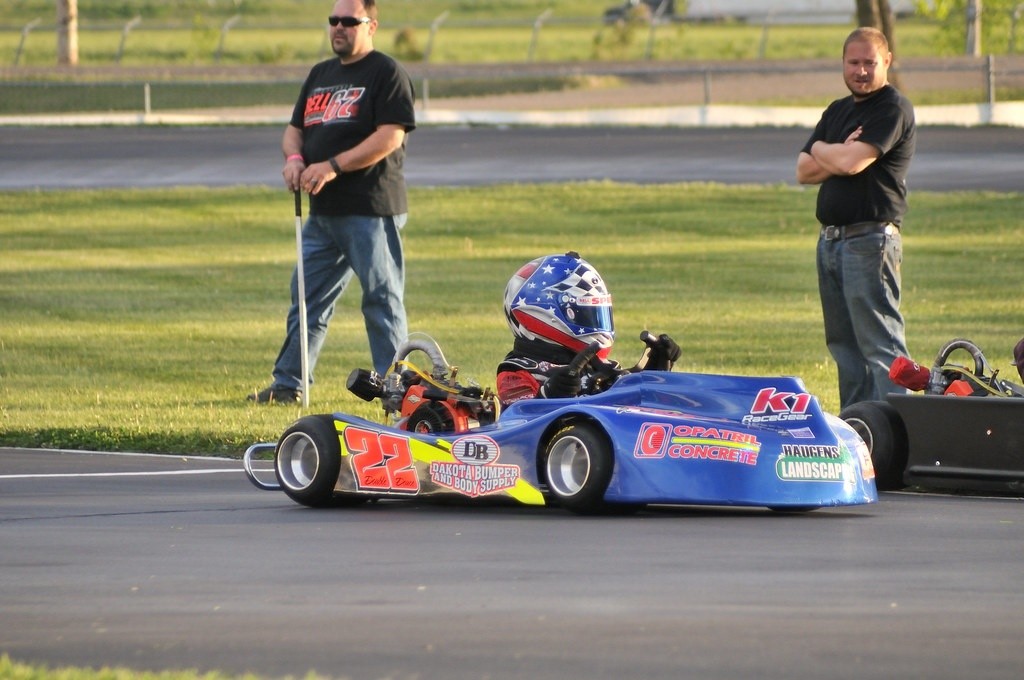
[542,375,582,400]
[652,334,681,372]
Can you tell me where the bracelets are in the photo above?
[327,157,343,176]
[285,154,304,161]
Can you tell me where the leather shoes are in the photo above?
[247,388,302,404]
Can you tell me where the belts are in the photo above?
[822,222,901,245]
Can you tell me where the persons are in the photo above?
[245,0,417,404]
[495,253,683,410]
[796,26,916,411]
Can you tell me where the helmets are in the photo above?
[502,255,614,361]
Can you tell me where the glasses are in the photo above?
[328,16,372,28]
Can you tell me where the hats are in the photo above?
[1011,337,1024,366]
